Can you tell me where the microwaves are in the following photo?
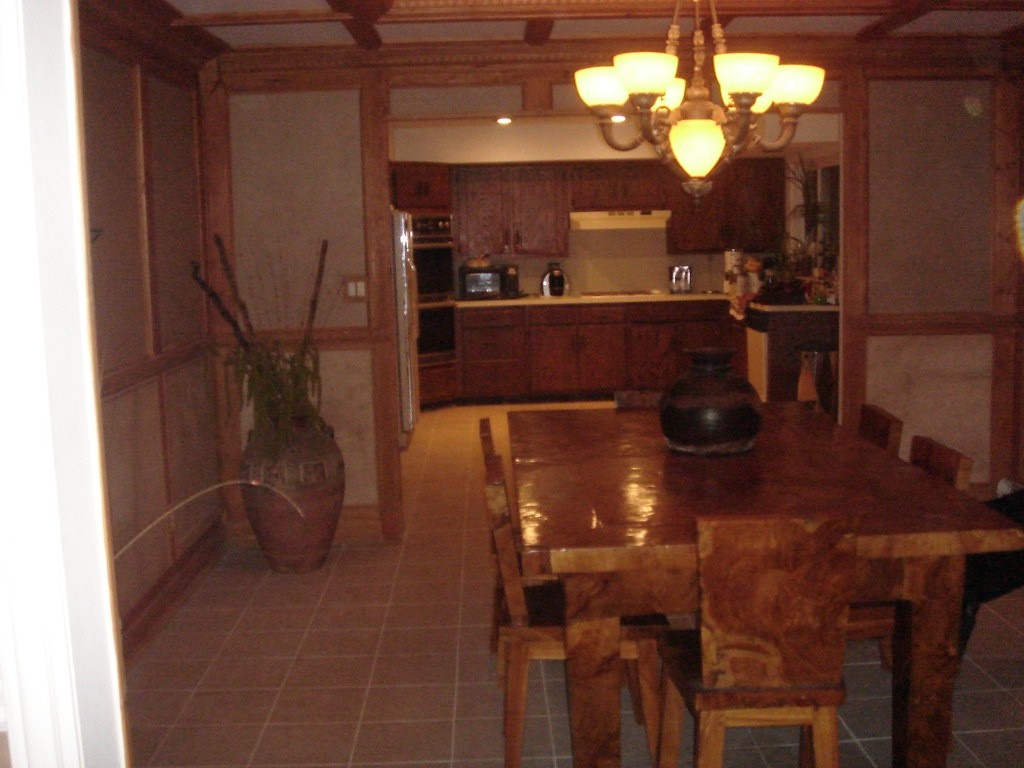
[459,263,518,300]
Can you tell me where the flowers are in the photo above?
[791,235,839,290]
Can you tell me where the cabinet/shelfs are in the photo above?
[419,300,729,403]
[390,158,782,259]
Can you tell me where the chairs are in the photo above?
[480,402,1024,767]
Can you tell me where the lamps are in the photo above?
[575,0,826,199]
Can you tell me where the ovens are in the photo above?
[410,241,458,370]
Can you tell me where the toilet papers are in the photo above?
[724,251,744,293]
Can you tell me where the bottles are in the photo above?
[722,248,744,294]
[658,347,763,454]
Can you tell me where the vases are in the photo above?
[809,268,830,305]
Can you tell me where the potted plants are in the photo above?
[233,340,348,570]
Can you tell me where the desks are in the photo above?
[744,302,841,401]
[501,401,1024,768]
